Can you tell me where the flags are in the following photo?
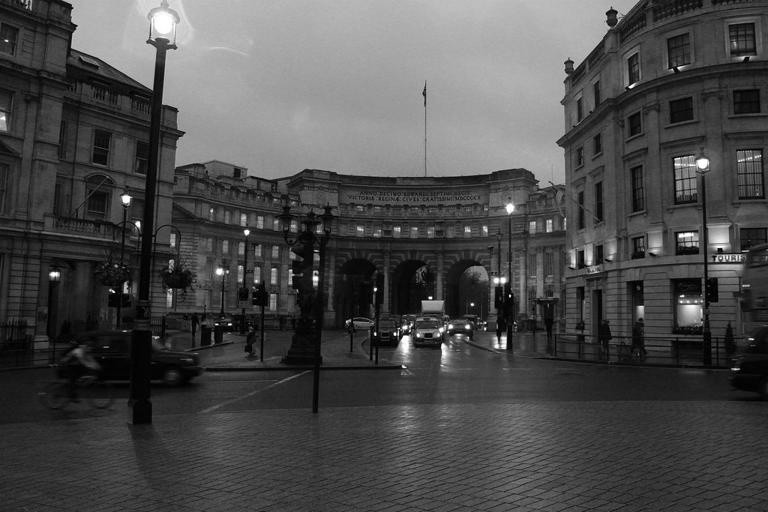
[421,82,426,108]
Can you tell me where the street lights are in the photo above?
[695,147,712,365]
[47,263,63,338]
[134,0,180,424]
[244,226,249,288]
[116,192,133,329]
[507,200,514,350]
[217,258,230,313]
[274,204,337,413]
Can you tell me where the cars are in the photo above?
[728,325,768,391]
[57,330,200,384]
[345,300,487,348]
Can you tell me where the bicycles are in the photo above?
[39,370,114,408]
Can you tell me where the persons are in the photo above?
[598,318,613,350]
[55,339,101,406]
[243,323,256,358]
[628,317,648,357]
